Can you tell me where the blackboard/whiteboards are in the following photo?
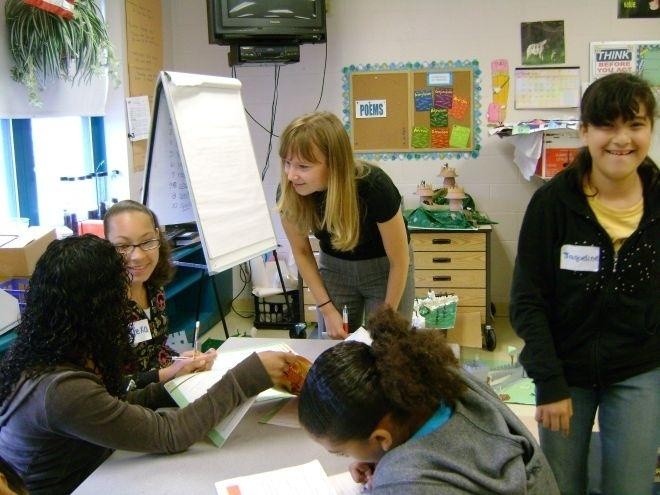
[160,69,278,278]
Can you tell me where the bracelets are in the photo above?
[316,299,333,308]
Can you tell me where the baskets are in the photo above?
[251,289,299,330]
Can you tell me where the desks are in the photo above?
[69,335,369,495]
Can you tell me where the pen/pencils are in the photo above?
[343,305,349,333]
[193,320,200,360]
[171,357,193,360]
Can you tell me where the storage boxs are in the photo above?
[1,225,58,285]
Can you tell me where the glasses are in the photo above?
[112,229,161,254]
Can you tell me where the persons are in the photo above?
[506,70,660,493]
[276,110,415,340]
[298,305,558,493]
[103,199,218,397]
[0,234,299,494]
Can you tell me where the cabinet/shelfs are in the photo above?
[409,227,497,351]
[299,236,321,330]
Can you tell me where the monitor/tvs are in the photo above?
[206,0,327,46]
